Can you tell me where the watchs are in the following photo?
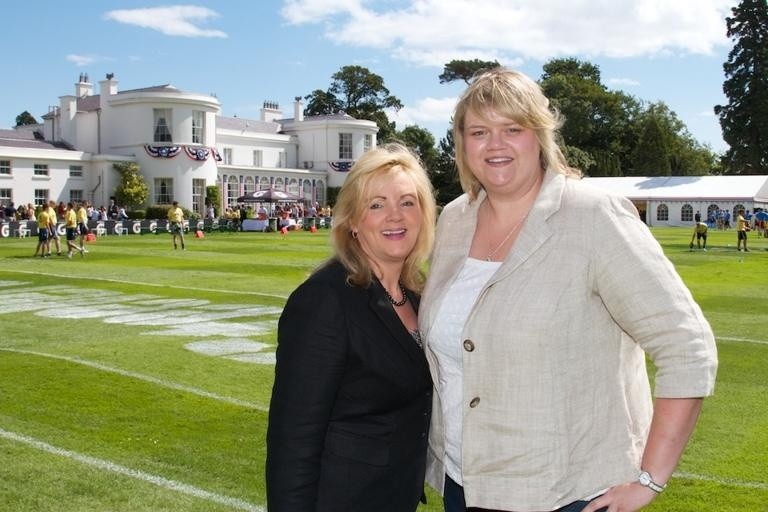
[637,467,667,494]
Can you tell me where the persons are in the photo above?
[417,69,719,512]
[258,205,267,220]
[0,199,130,259]
[207,203,214,222]
[223,204,256,224]
[167,202,185,251]
[688,207,768,251]
[264,147,434,511]
[206,203,216,219]
[269,201,334,227]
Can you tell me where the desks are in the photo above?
[242,219,269,232]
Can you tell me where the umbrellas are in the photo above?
[236,186,304,217]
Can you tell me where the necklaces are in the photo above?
[383,278,407,307]
[484,203,532,262]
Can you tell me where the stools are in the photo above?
[14,229,31,239]
[119,227,128,236]
[90,228,107,236]
